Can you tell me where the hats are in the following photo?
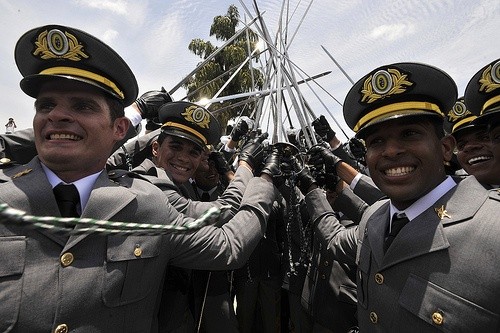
[156,100,220,152]
[341,60,460,134]
[440,57,500,136]
[12,22,139,107]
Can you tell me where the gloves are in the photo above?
[238,131,270,171]
[310,114,336,144]
[261,142,343,195]
[135,89,173,121]
[348,135,367,160]
[230,119,249,142]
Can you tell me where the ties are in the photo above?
[55,184,80,218]
[383,213,409,253]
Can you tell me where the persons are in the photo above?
[5,118,17,132]
[0,90,168,169]
[104,123,164,172]
[288,61,500,333]
[131,100,268,227]
[464,56,500,165]
[0,21,281,333]
[444,96,500,194]
[178,115,387,333]
